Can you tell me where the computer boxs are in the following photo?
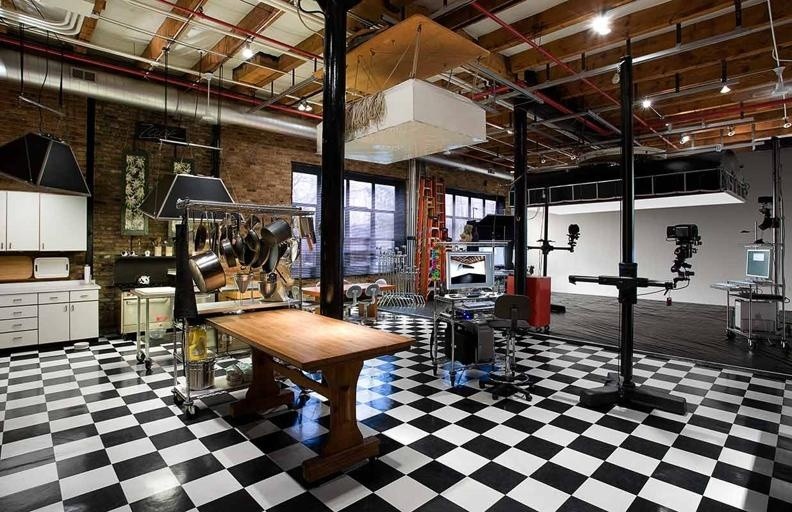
[445,318,495,364]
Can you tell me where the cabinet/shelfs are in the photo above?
[0,280,101,351]
[432,240,516,386]
[117,290,175,337]
[0,190,89,253]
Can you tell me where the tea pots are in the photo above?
[138,275,151,284]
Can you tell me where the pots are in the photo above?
[189,211,299,293]
[182,350,216,389]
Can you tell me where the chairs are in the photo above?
[315,277,397,317]
[479,293,534,401]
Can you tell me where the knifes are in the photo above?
[298,216,318,251]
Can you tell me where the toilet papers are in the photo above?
[84,266,90,284]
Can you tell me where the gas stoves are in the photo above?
[115,281,170,297]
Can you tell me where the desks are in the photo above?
[204,306,415,484]
[301,282,397,299]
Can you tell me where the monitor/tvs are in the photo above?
[746,249,771,282]
[446,251,494,297]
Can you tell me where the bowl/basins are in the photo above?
[147,327,167,340]
[226,369,242,386]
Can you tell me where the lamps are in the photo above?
[680,118,791,144]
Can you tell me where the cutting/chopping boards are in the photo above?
[134,285,176,295]
[0,256,33,282]
[33,257,69,278]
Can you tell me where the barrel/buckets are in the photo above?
[183,357,215,390]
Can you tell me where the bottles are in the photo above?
[136,235,176,257]
[497,276,504,293]
[83,264,91,284]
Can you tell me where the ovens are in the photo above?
[114,297,172,333]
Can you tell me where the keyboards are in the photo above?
[462,300,495,308]
